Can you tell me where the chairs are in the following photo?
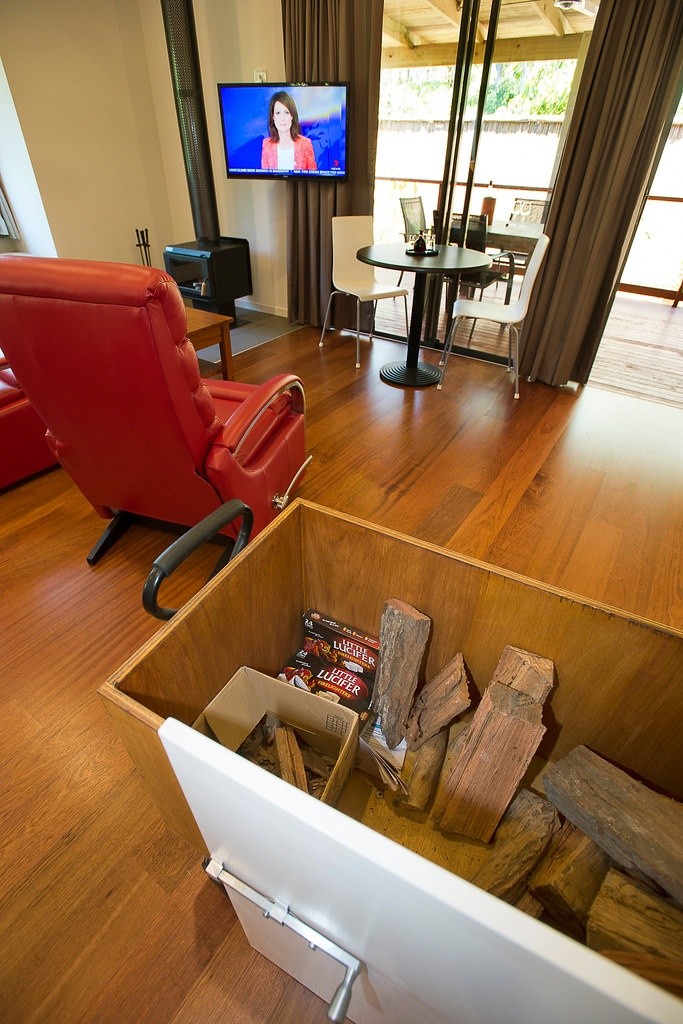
[437,234,550,400]
[0,254,313,620]
[496,198,550,290]
[319,216,409,368]
[425,209,515,339]
[393,196,432,299]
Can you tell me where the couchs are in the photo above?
[0,349,57,493]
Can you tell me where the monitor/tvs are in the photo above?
[217,82,349,182]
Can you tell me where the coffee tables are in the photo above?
[185,306,236,383]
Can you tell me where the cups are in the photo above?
[481,197,496,225]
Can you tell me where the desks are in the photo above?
[355,242,492,389]
[428,217,545,296]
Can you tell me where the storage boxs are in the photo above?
[190,608,380,809]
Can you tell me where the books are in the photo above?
[279,607,410,794]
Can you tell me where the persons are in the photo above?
[260,91,317,171]
